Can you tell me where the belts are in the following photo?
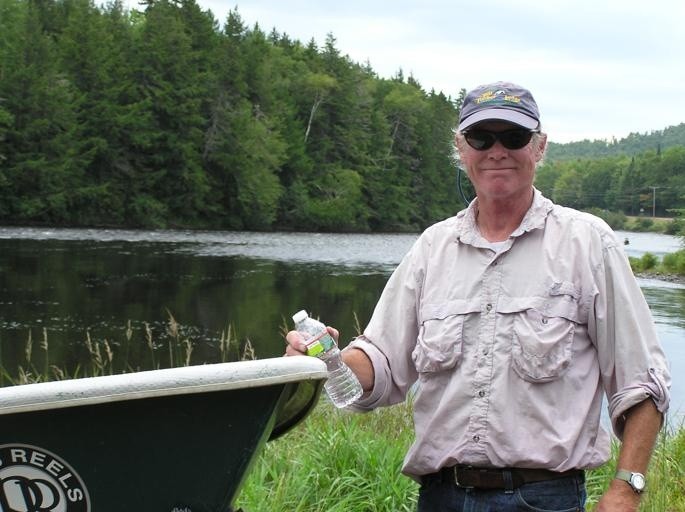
[419,464,585,490]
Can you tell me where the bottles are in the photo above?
[291,309,363,409]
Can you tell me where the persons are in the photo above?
[284,81,671,512]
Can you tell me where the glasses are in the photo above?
[461,129,540,150]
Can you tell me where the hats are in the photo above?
[458,81,541,133]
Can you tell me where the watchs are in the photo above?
[614,469,646,492]
